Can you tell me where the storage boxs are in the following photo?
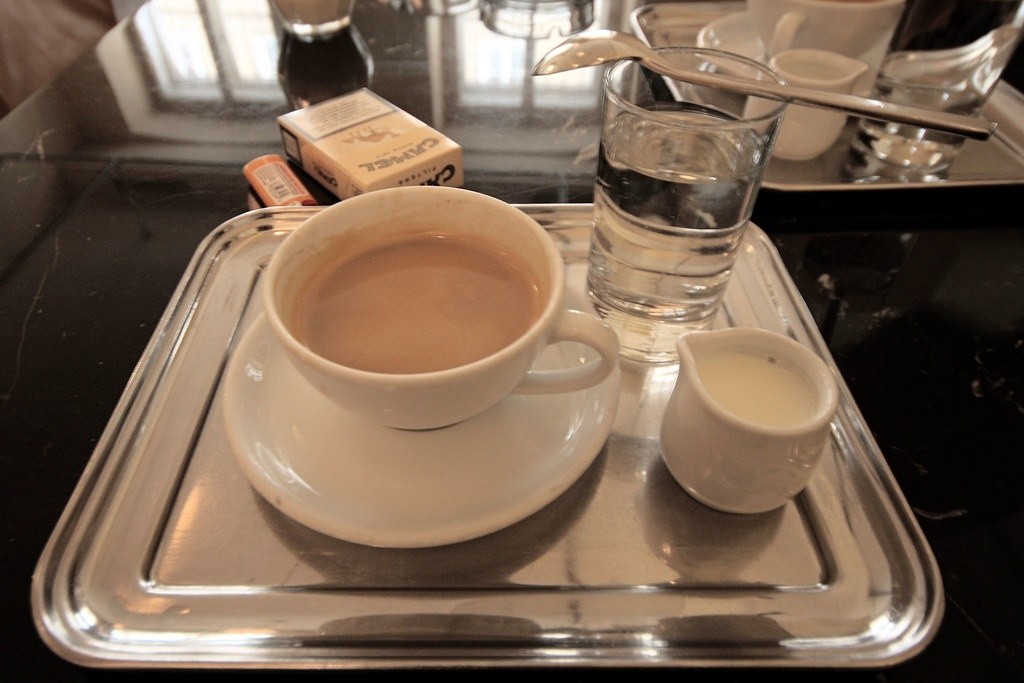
[277,86,467,204]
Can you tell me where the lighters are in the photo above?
[242,153,319,207]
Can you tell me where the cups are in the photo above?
[748,0,905,57]
[263,185,622,433]
[845,0,1024,177]
[742,48,870,162]
[381,0,596,40]
[530,28,991,142]
[270,0,355,40]
[659,327,839,514]
[582,45,795,369]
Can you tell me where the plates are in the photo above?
[220,312,623,546]
[696,11,777,82]
